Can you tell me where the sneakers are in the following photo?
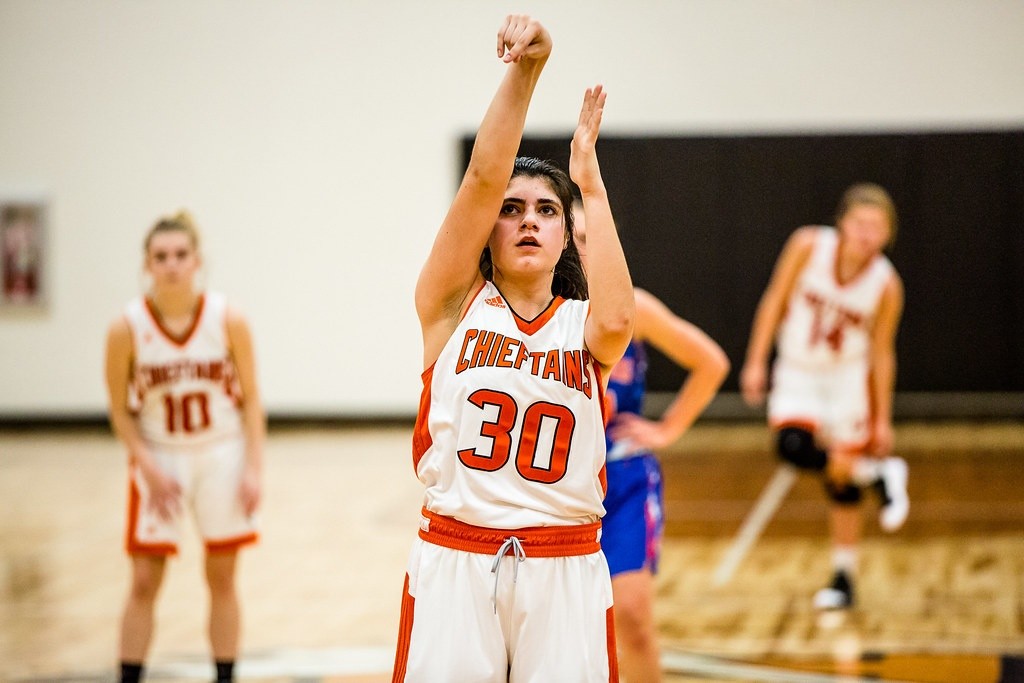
[873,455,913,535]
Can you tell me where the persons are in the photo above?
[384,12,635,682]
[557,196,730,683]
[108,214,260,682]
[738,184,910,609]
